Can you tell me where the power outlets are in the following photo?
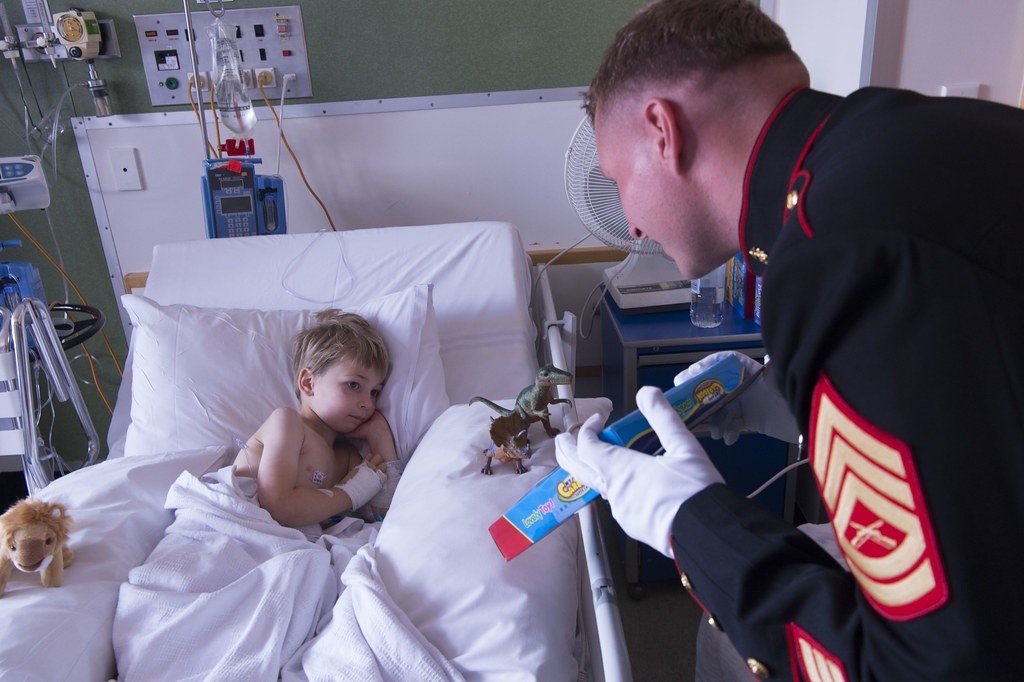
[131,3,314,108]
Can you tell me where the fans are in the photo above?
[563,113,727,320]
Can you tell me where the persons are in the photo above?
[553,0,1024,682]
[123,306,405,681]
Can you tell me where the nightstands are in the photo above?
[599,287,763,605]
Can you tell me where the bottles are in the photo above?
[688,260,725,330]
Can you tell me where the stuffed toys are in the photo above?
[0,499,74,599]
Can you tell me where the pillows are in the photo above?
[118,290,452,470]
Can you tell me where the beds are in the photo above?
[0,220,635,681]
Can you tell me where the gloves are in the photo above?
[555,383,728,560]
[672,350,772,447]
